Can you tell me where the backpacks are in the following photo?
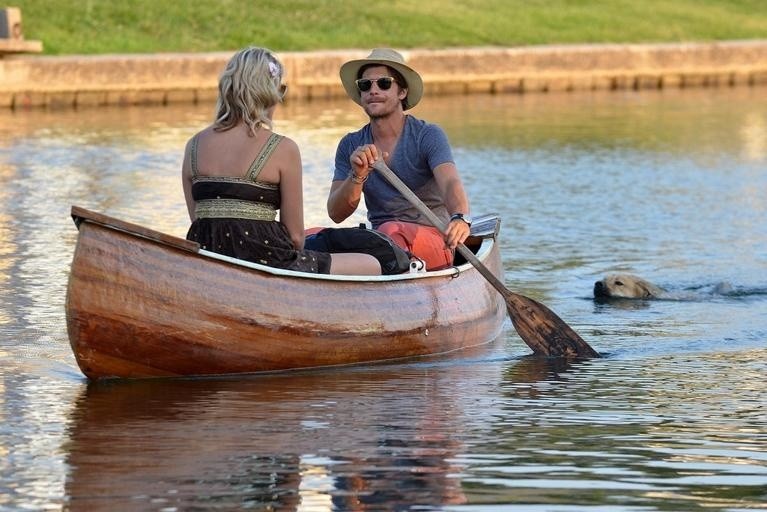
[301,226,409,275]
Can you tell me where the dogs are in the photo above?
[593,272,736,304]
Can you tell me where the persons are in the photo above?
[303,48,473,272]
[180,46,383,277]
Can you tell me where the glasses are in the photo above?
[354,76,400,93]
[276,81,287,99]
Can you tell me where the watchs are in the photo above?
[450,211,472,227]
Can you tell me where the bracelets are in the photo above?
[349,169,369,184]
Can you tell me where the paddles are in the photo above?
[365,148,603,358]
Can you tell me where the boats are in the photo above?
[65,206,505,383]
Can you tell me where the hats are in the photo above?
[336,47,424,114]
